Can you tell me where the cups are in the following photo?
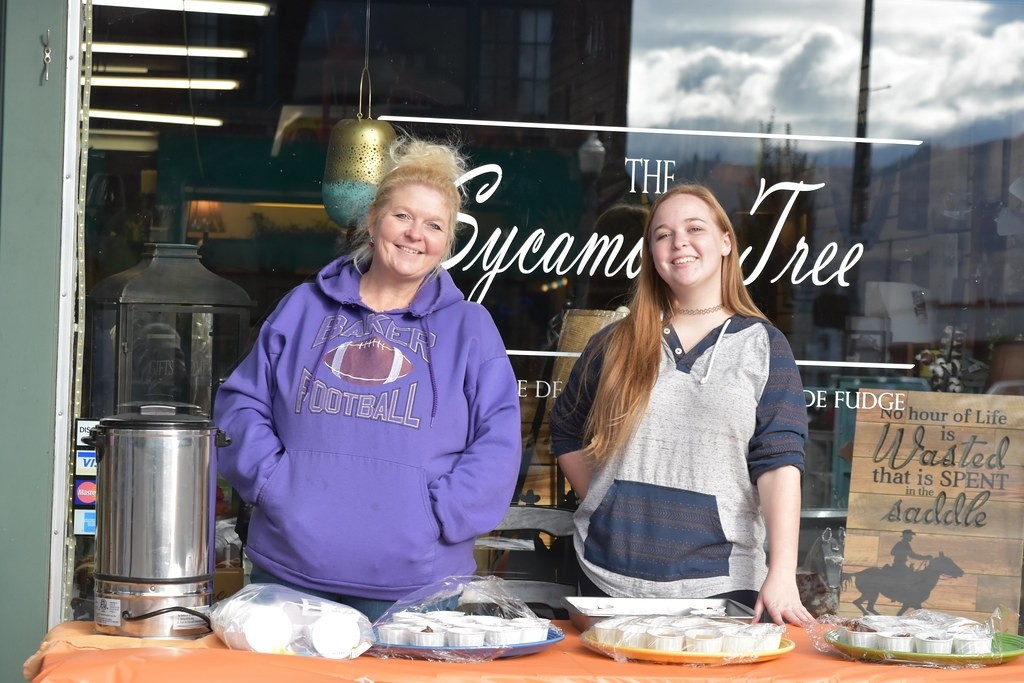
[213,601,361,659]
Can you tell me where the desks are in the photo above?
[22,621,1024,683]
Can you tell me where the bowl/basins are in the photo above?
[594,615,783,653]
[377,610,551,646]
[838,615,992,655]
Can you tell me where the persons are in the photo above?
[553,185,815,628]
[587,204,652,311]
[215,138,521,623]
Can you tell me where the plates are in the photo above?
[371,628,566,658]
[583,631,795,664]
[824,629,1024,665]
